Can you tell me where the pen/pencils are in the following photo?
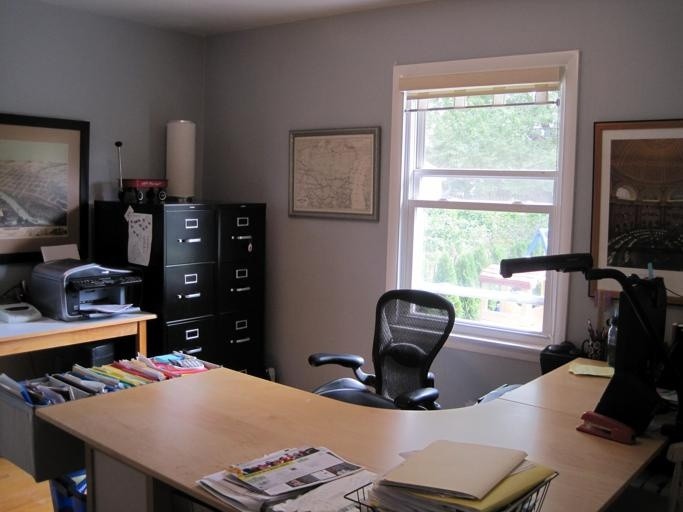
[586,319,604,339]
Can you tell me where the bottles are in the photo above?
[607,315,619,367]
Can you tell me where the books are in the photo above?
[367,440,555,512]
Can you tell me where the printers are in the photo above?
[29,257,142,320]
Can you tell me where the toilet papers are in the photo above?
[166,121,196,198]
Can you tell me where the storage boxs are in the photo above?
[0,353,219,511]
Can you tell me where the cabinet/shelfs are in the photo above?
[93,197,266,384]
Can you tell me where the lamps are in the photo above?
[499,253,668,434]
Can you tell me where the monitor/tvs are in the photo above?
[591,275,667,433]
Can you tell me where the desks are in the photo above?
[0,309,158,359]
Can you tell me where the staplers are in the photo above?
[576,411,636,444]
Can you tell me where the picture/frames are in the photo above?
[286,125,381,223]
[0,111,90,267]
[585,118,682,303]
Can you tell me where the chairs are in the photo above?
[306,288,454,410]
[32,356,679,512]
